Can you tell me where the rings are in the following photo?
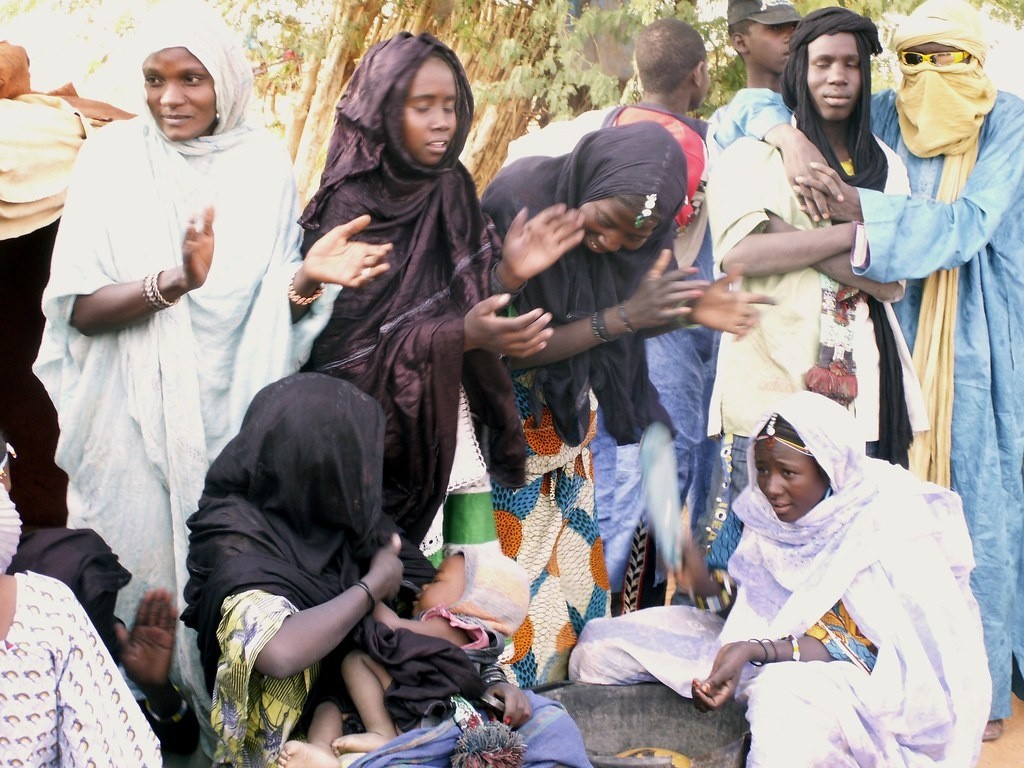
[361,267,374,282]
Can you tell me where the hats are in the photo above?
[445,540,531,636]
[728,0,802,25]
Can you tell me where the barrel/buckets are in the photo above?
[529,680,751,768]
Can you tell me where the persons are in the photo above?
[0,0,1024,768]
[568,391,991,768]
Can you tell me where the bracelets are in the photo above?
[592,310,618,343]
[288,272,326,305]
[781,635,800,661]
[141,270,178,311]
[761,639,778,662]
[481,665,508,683]
[354,581,375,615]
[145,683,187,724]
[619,301,635,335]
[749,639,768,666]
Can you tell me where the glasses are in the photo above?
[900,50,971,67]
